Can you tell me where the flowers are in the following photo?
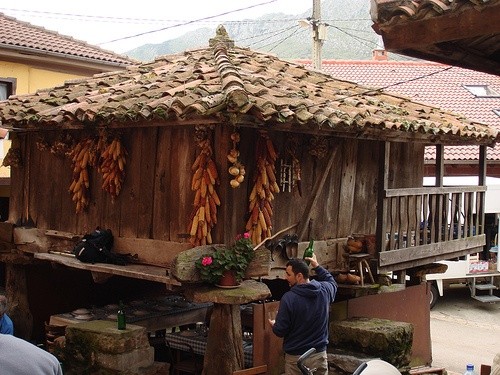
[196,233,257,287]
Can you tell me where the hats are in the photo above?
[0,296,6,303]
[489,247,498,252]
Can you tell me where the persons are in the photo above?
[0,334,62,375]
[0,295,13,335]
[268,253,337,375]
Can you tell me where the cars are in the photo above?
[386,198,480,310]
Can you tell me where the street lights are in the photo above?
[296,19,330,71]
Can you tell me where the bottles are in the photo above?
[117,305,126,330]
[465,365,475,375]
[303,238,314,266]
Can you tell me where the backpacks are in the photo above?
[74,227,115,264]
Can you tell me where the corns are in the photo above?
[68,137,124,215]
[297,179,302,197]
[245,137,280,249]
[189,140,220,249]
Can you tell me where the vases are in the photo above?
[219,270,239,286]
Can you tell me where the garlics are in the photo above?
[35,140,67,155]
[227,132,245,187]
